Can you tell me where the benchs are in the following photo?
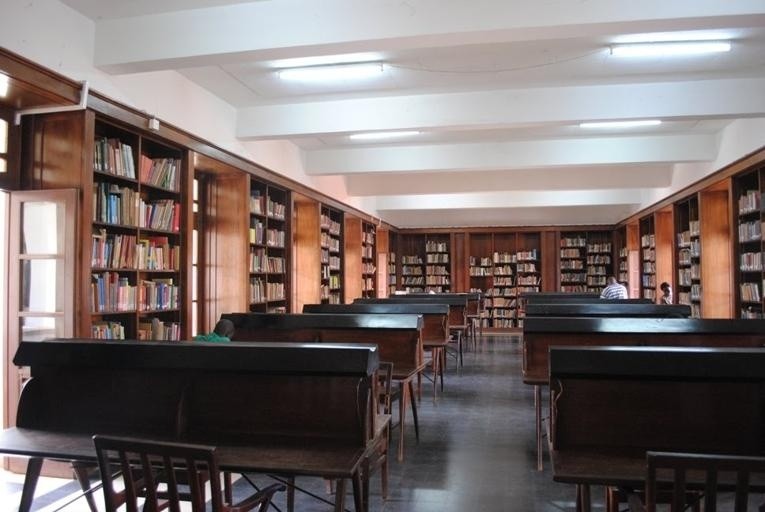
[522,292,765,471]
[544,346,765,511]
[0,340,395,512]
[205,293,485,511]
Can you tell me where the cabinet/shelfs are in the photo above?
[377,226,618,332]
[210,171,380,330]
[28,110,190,346]
[618,160,764,323]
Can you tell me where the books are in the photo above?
[362,232,375,299]
[320,215,341,304]
[388,236,628,329]
[91,135,182,341]
[641,190,765,320]
[250,191,287,314]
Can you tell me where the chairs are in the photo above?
[92,433,287,512]
[643,448,765,511]
